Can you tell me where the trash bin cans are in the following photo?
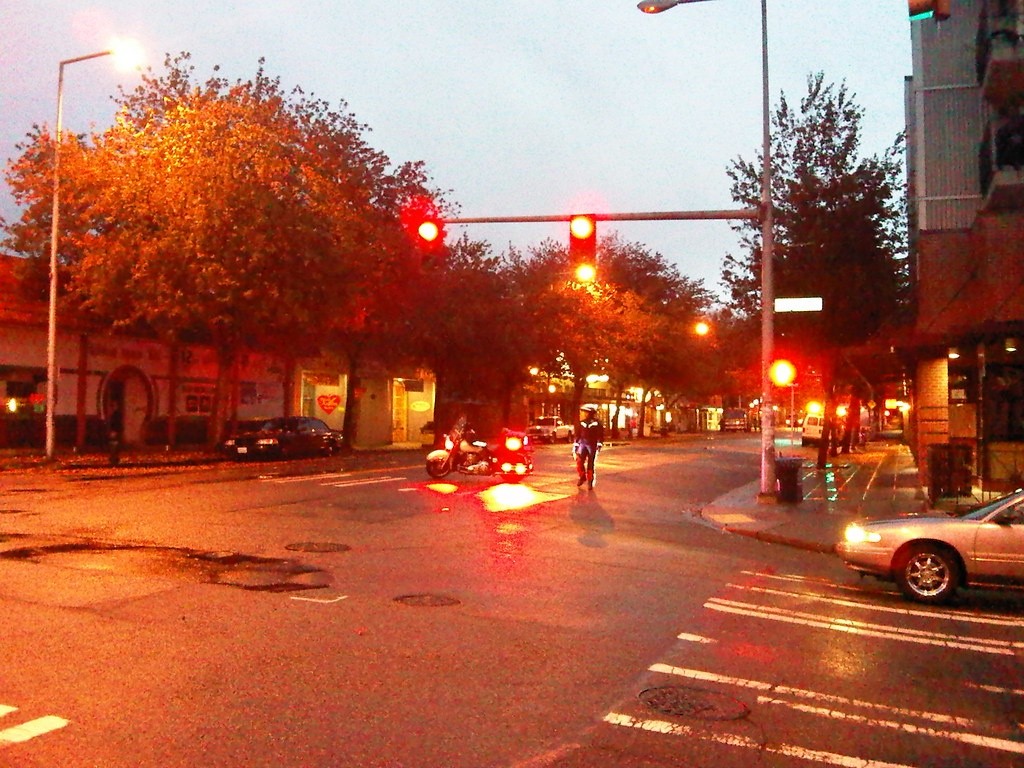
[774,455,804,504]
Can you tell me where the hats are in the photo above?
[579,407,597,413]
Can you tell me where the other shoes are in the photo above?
[588,479,592,489]
[577,477,586,487]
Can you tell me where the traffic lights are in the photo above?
[417,218,442,277]
[570,215,596,285]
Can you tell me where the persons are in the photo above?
[573,403,604,490]
[717,418,762,432]
[107,400,123,465]
[611,415,695,434]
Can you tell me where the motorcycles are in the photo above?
[427,412,536,482]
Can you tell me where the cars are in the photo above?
[223,415,343,461]
[835,490,1024,606]
[525,417,575,444]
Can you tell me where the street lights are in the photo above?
[638,0,783,507]
[46,43,144,454]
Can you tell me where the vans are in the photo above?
[802,415,846,447]
